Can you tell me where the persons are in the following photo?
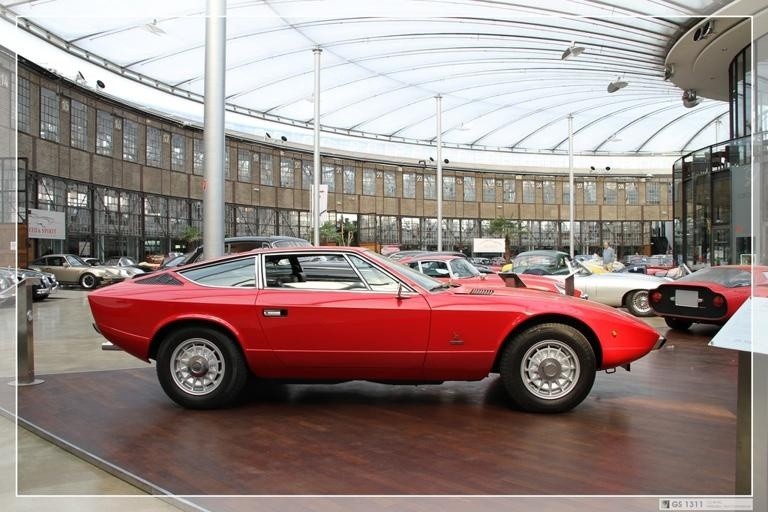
[602,240,615,272]
[159,251,173,265]
